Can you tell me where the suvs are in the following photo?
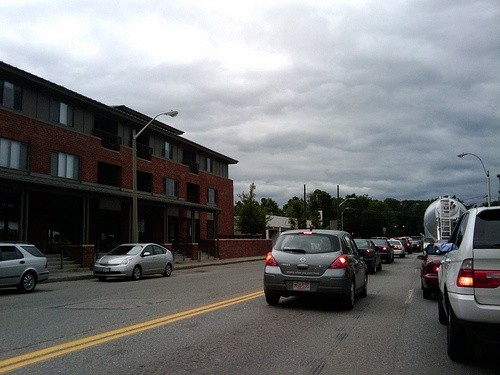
[0,241,48,293]
[438,205,500,364]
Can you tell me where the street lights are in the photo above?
[458,153,491,207]
[132,110,179,243]
[337,197,355,229]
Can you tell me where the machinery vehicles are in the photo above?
[423,194,467,244]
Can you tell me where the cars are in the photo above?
[418,242,446,301]
[92,243,174,281]
[353,236,424,273]
[264,229,368,310]
